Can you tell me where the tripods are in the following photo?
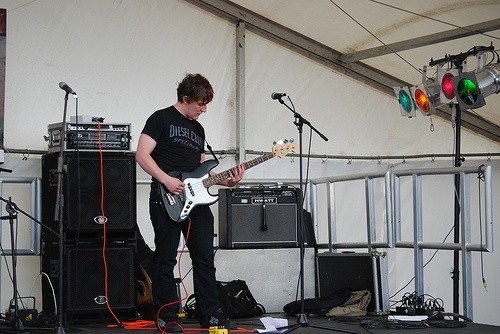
[0,196,65,334]
[276,99,355,334]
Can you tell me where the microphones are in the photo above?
[271,92,287,100]
[59,82,77,96]
[261,203,268,231]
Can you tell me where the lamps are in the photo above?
[392,41,500,128]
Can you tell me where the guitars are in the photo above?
[158,138,299,223]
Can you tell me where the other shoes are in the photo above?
[200,308,230,329]
[156,318,184,332]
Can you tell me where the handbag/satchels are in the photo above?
[217,279,265,318]
[326,290,371,316]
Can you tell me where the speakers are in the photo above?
[43,238,137,313]
[42,152,137,239]
[226,190,301,248]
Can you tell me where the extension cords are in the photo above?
[387,315,428,321]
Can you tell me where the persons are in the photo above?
[135,74,244,332]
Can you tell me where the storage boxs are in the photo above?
[316,250,389,314]
